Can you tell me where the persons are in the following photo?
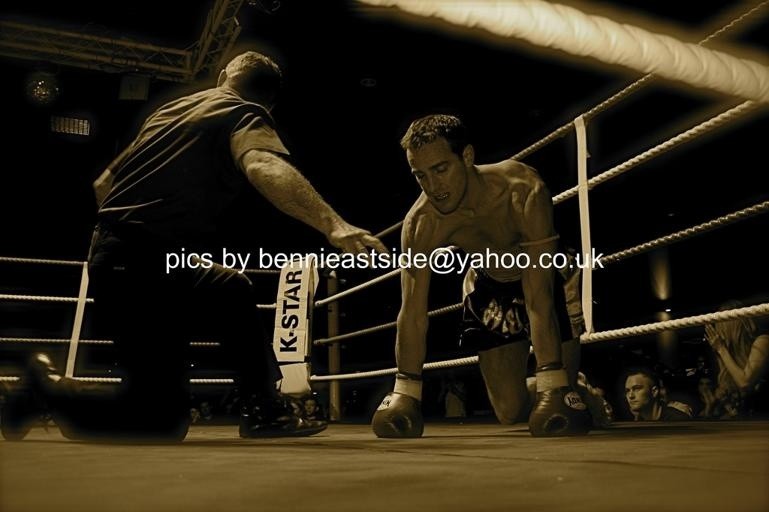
[0,50,392,447]
[0,292,768,426]
[374,115,613,438]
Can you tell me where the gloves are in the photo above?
[372,374,424,437]
[529,364,601,435]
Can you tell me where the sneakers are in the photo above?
[240,401,328,438]
[1,354,57,440]
